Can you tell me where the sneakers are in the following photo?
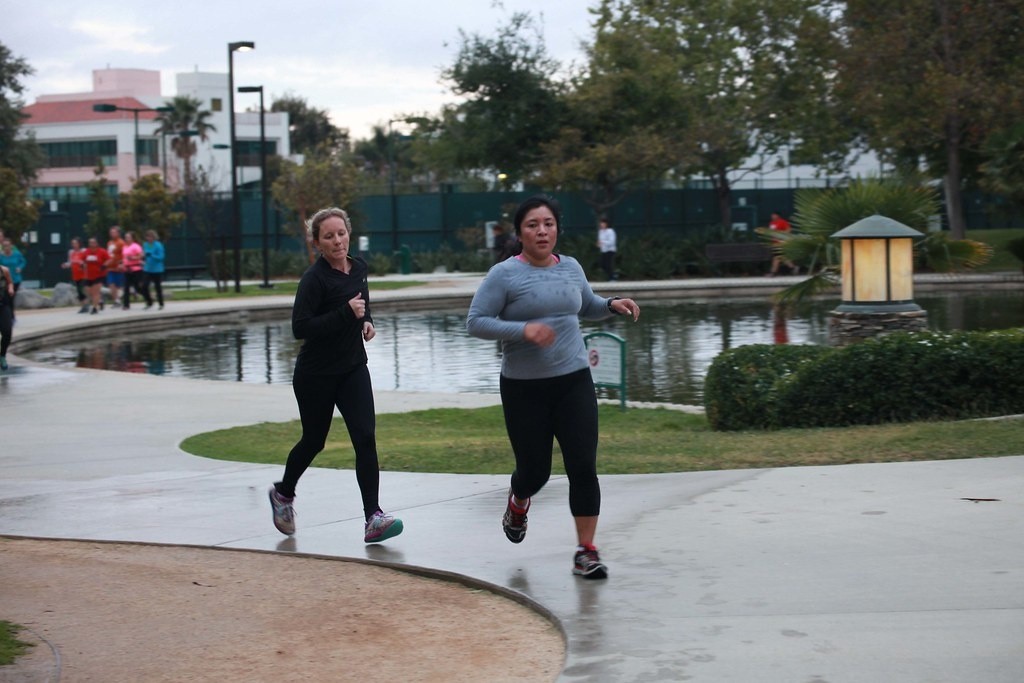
[269,489,295,535]
[573,545,608,578]
[0,356,8,371]
[365,512,403,543]
[77,300,165,315]
[502,487,530,543]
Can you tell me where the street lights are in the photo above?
[93,104,173,179]
[228,41,255,294]
[238,87,268,285]
[162,129,197,186]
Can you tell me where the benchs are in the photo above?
[705,243,776,276]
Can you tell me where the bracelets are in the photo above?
[608,296,621,315]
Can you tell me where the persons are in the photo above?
[0,231,27,369]
[765,212,802,276]
[491,225,512,263]
[465,198,641,577]
[596,218,623,281]
[268,207,404,543]
[62,225,165,315]
